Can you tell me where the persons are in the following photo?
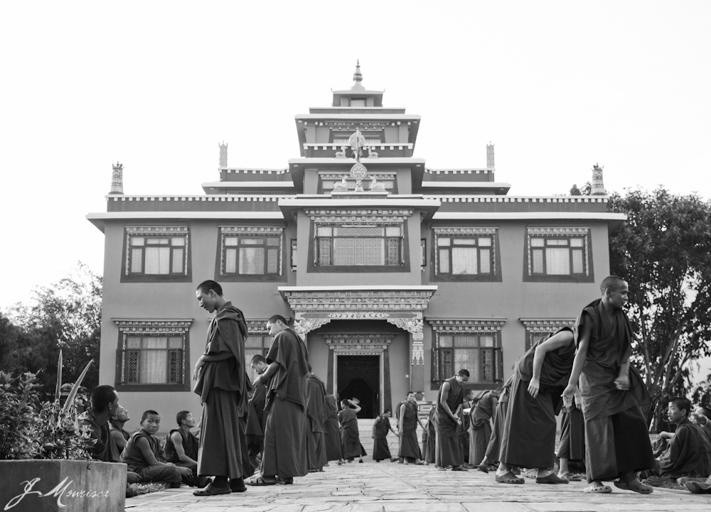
[81,273,367,496]
[570,184,583,196]
[371,276,710,495]
[581,182,591,196]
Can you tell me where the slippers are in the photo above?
[536,472,569,484]
[584,485,612,492]
[614,478,653,494]
[496,473,524,484]
[193,476,293,496]
